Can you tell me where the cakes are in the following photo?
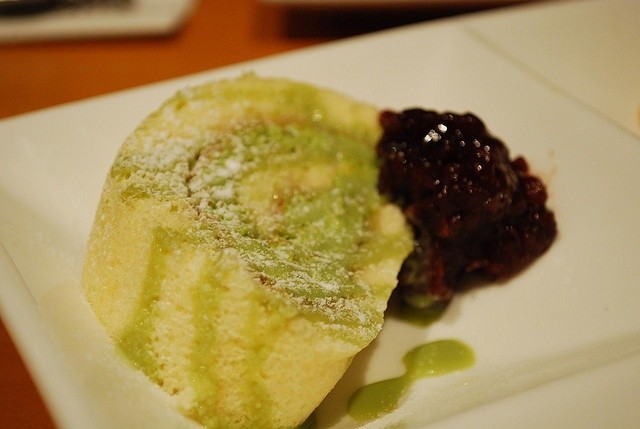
[83,72,418,428]
